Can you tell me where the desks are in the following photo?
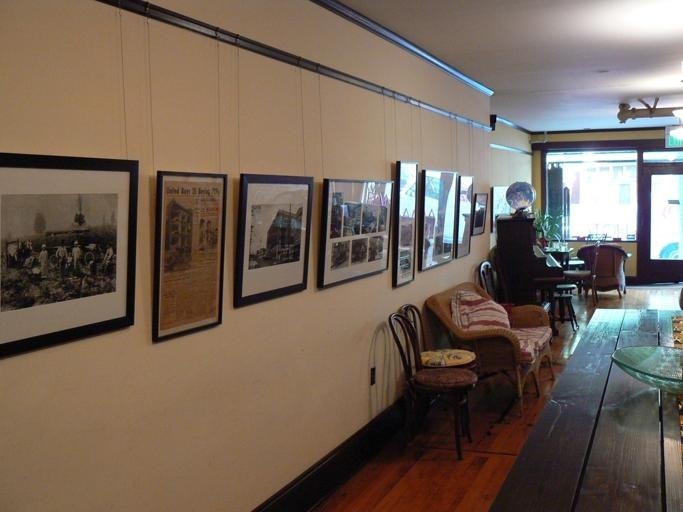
[543,247,574,294]
[490,308,683,512]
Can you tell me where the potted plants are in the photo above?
[536,209,563,249]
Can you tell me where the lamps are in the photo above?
[617,98,683,124]
[665,109,683,148]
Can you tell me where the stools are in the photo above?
[552,294,579,331]
[556,284,577,323]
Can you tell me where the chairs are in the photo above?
[563,233,607,294]
[399,303,480,437]
[563,241,600,307]
[389,313,478,461]
[478,260,559,337]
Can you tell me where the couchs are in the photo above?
[425,282,555,417]
[577,244,628,299]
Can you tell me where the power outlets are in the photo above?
[370,367,376,385]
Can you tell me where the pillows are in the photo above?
[451,289,510,331]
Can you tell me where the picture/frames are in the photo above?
[418,169,458,273]
[0,152,139,359]
[491,186,511,233]
[471,192,488,236]
[392,159,419,288]
[317,178,394,290]
[455,176,473,259]
[151,170,228,344]
[233,173,314,309]
